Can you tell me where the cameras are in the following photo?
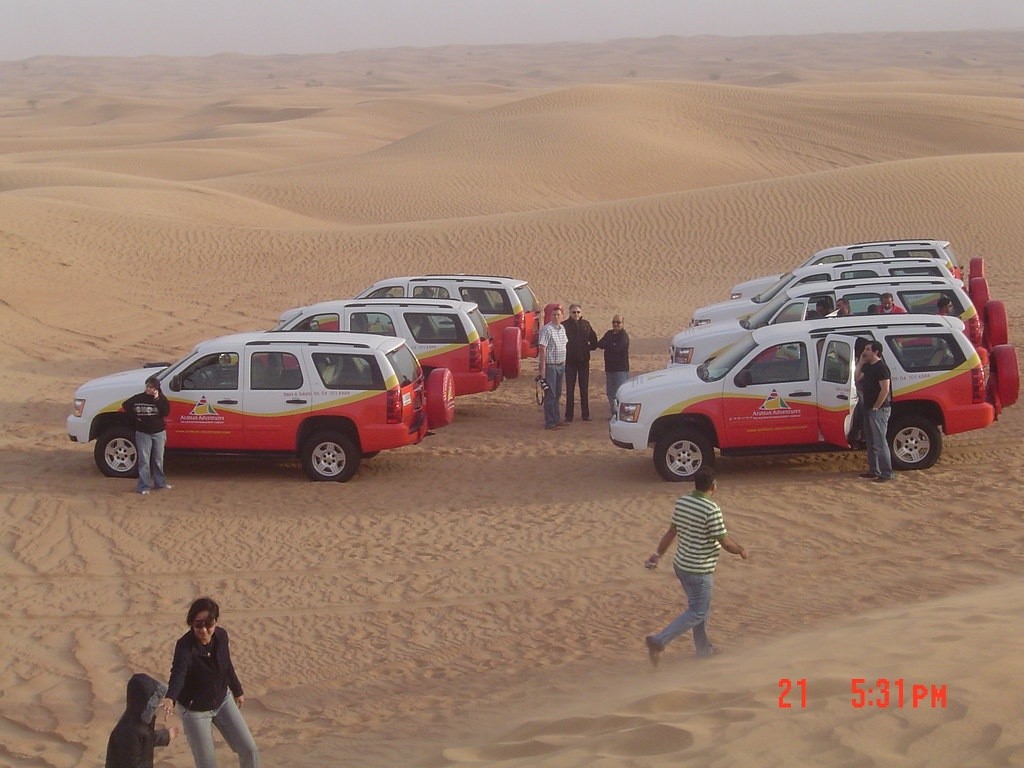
[535,374,550,392]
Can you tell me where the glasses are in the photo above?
[190,619,216,628]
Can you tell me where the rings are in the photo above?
[168,706,170,710]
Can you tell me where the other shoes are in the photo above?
[859,473,894,483]
[545,422,570,430]
[161,484,174,489]
[646,635,664,669]
[697,646,724,658]
[141,490,150,495]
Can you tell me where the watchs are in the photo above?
[872,407,879,412]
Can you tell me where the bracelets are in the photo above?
[655,552,662,558]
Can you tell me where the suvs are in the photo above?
[609,312,1020,482]
[730,238,985,300]
[687,255,990,331]
[65,329,457,484]
[279,273,564,360]
[667,274,1009,370]
[191,296,522,398]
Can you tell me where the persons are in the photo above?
[854,341,896,483]
[122,377,175,496]
[934,296,954,316]
[560,303,597,422]
[105,672,180,768]
[835,296,856,317]
[643,468,751,668]
[538,308,569,432]
[597,315,630,421]
[159,597,260,768]
[816,300,830,320]
[874,291,906,314]
